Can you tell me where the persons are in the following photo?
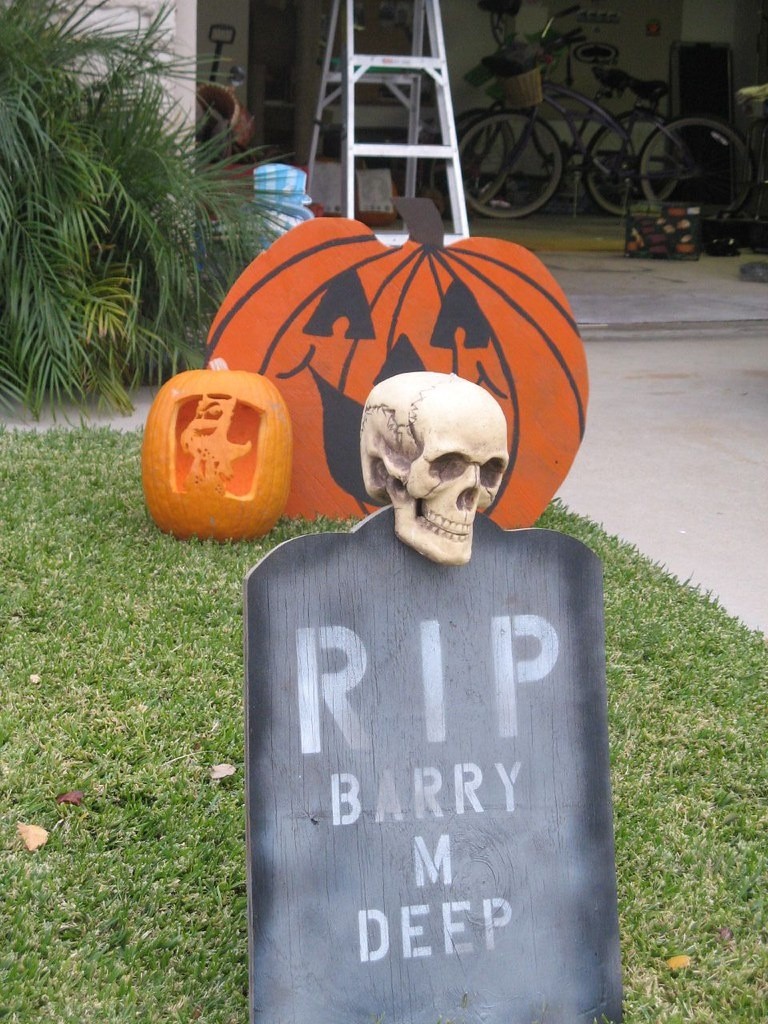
[306,123,345,215]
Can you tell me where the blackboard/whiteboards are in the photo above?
[246,501,625,1024]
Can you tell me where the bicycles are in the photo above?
[420,0,760,221]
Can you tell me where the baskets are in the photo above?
[502,63,544,110]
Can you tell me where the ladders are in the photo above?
[306,0,471,245]
